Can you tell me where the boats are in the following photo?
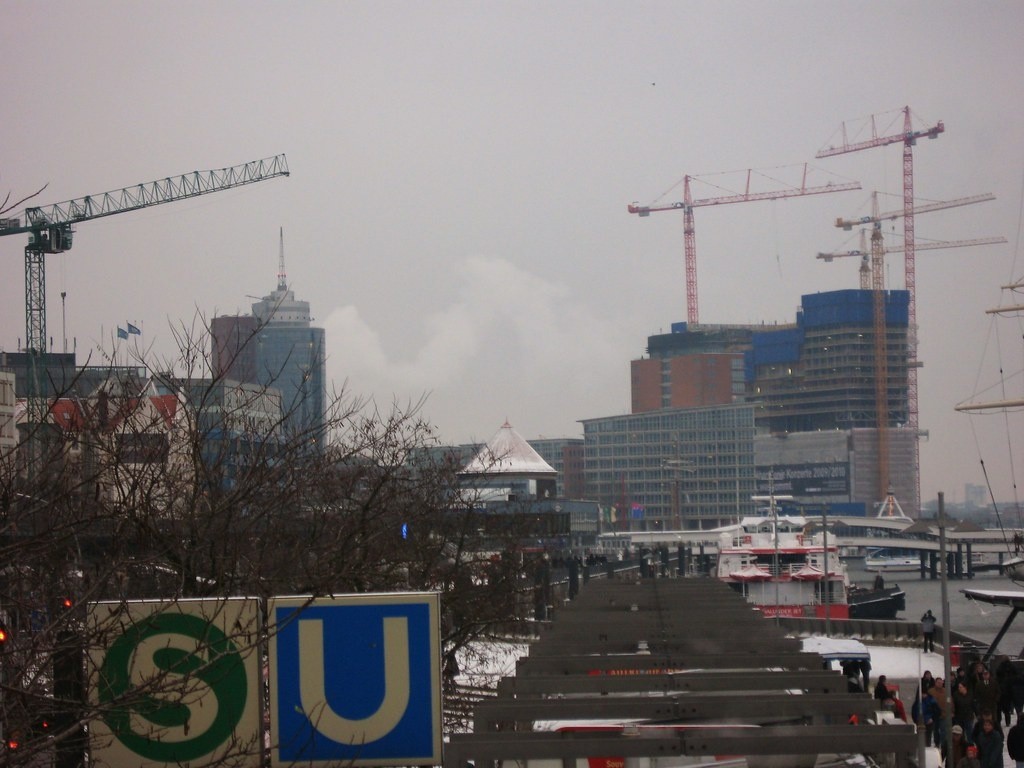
[710,271,1024,664]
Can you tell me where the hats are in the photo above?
[952,724,964,736]
[982,667,992,679]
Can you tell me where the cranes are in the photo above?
[624,161,862,331]
[811,189,1009,499]
[812,103,1010,517]
[1,153,293,588]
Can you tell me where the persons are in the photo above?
[841,655,1024,768]
[921,609,936,654]
